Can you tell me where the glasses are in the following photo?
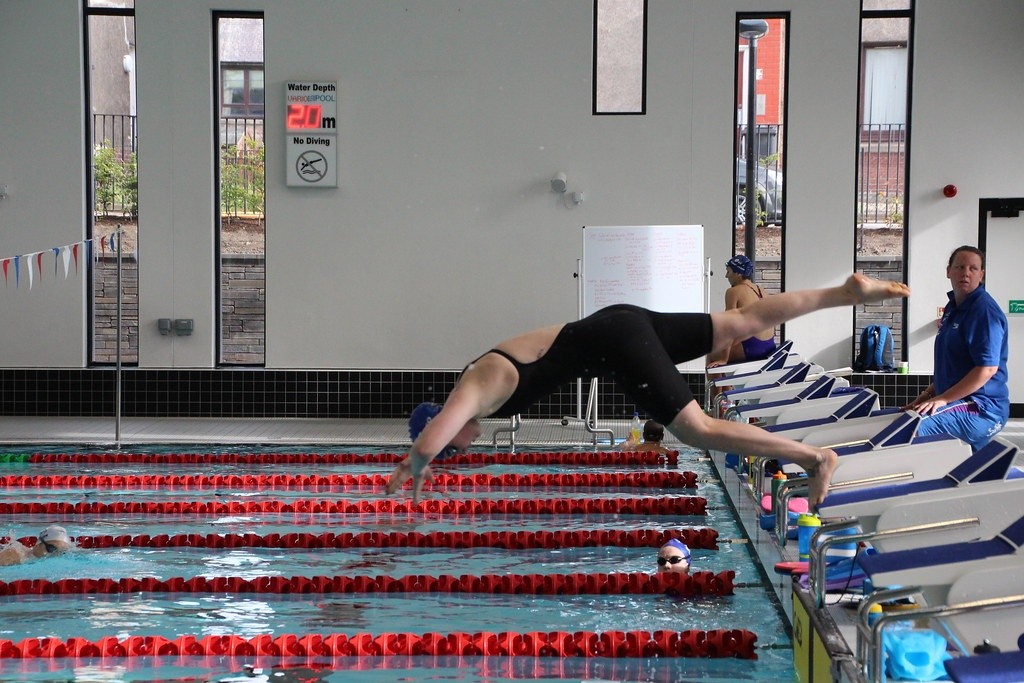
[446,446,465,457]
[725,262,728,267]
[657,556,690,566]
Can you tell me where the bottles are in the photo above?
[631,412,642,445]
[858,598,884,631]
[748,455,761,483]
[771,470,790,514]
[798,513,822,562]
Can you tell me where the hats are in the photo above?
[729,255,754,276]
[408,402,446,461]
[663,539,692,566]
[644,420,664,436]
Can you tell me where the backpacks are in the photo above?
[854,324,895,373]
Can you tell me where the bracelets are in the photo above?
[921,390,932,398]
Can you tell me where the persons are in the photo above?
[657,538,692,575]
[898,246,1010,445]
[1,526,74,567]
[384,272,910,514]
[616,418,670,454]
[705,254,777,417]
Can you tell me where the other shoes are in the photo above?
[759,511,799,529]
[760,495,809,514]
